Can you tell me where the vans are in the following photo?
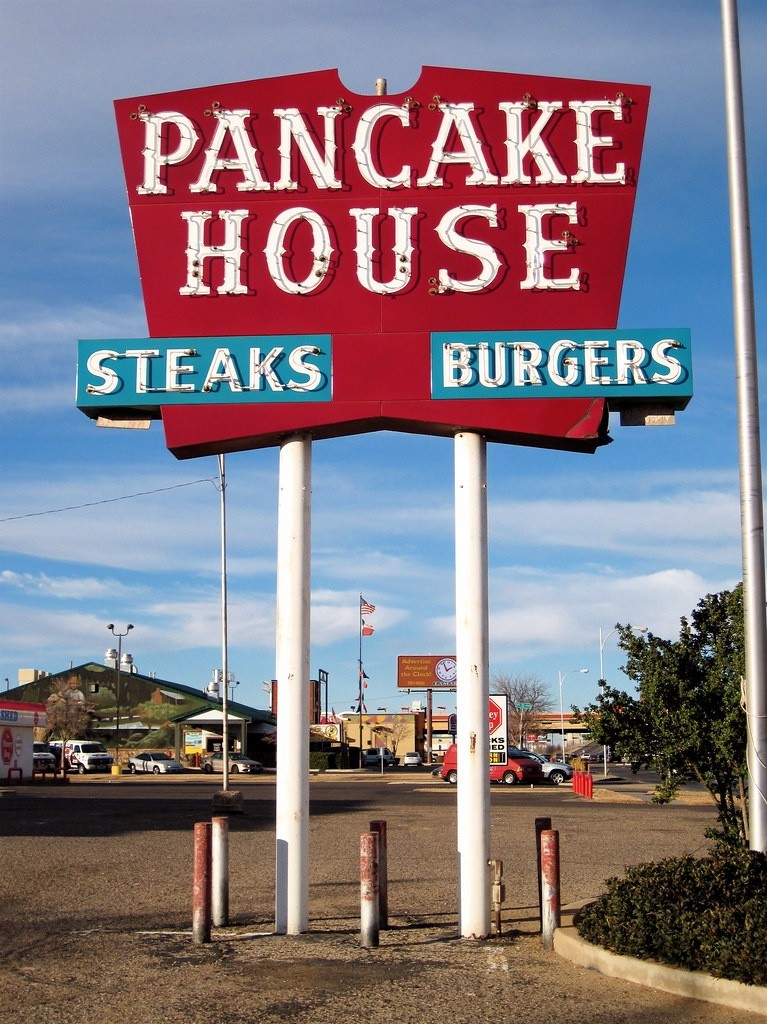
[49,739,113,775]
[364,748,396,767]
[441,743,545,784]
[32,741,57,773]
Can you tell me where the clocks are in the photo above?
[435,658,457,682]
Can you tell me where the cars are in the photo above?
[403,751,422,767]
[199,751,264,775]
[521,750,574,786]
[555,752,603,764]
[127,751,184,775]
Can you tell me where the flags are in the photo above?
[361,619,375,637]
[360,596,375,614]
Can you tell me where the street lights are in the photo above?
[558,669,589,764]
[106,623,134,776]
[598,625,648,778]
